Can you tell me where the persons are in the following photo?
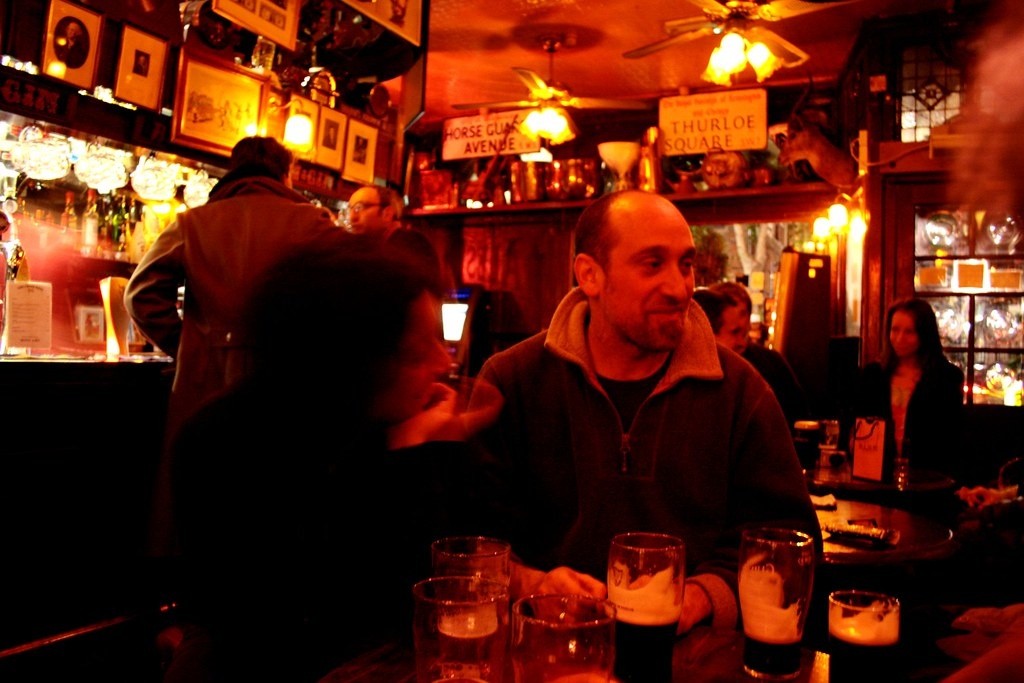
[967,481,1024,511]
[167,243,502,683]
[872,296,965,482]
[692,281,811,439]
[124,135,335,619]
[465,187,828,654]
[347,183,443,340]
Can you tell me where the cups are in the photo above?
[510,592,616,683]
[737,528,816,683]
[606,532,686,683]
[430,536,510,603]
[412,576,510,683]
[793,421,822,473]
[827,591,899,682]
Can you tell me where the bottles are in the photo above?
[62,187,134,261]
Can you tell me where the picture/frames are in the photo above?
[949,258,990,294]
[346,0,423,45]
[290,94,321,162]
[340,116,381,187]
[212,1,303,51]
[918,266,948,287]
[113,20,171,111]
[312,107,349,171]
[990,269,1022,291]
[41,1,105,90]
[169,46,271,156]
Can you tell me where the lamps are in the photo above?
[808,181,869,239]
[512,110,582,143]
[704,34,779,84]
[269,98,316,153]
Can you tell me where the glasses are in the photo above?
[345,201,382,218]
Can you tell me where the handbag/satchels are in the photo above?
[851,416,895,483]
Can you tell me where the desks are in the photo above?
[810,448,955,490]
[809,498,954,571]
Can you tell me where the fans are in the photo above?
[620,0,831,68]
[449,33,647,109]
